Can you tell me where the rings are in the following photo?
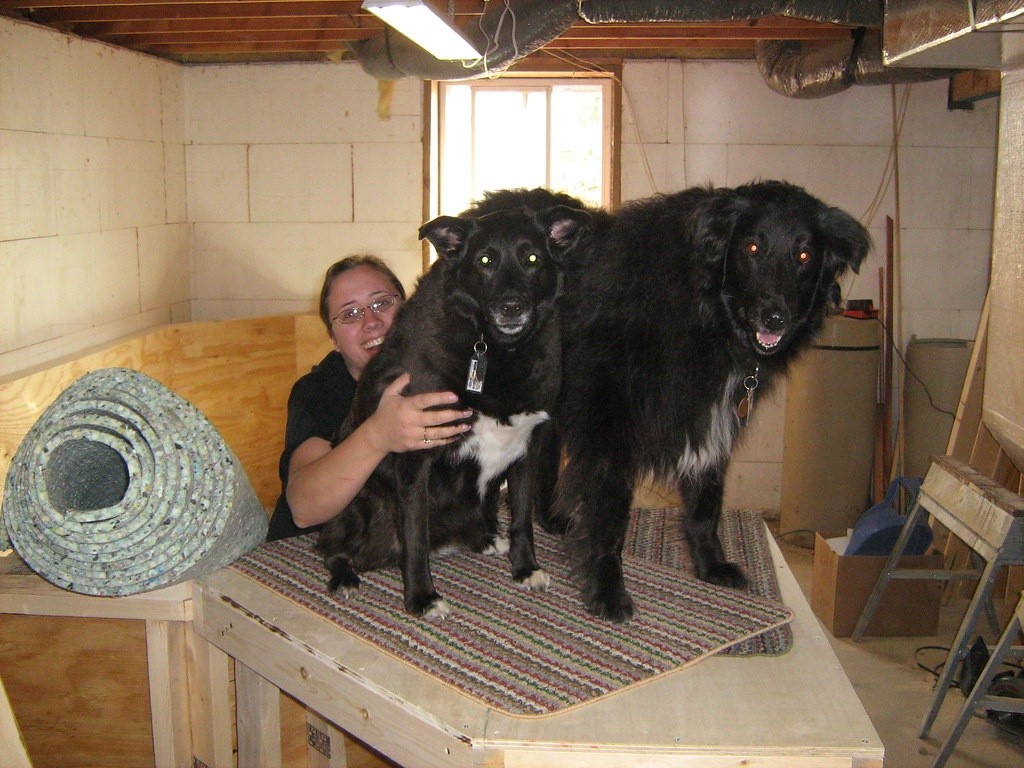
[423,426,431,443]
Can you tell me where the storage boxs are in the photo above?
[810,529,945,638]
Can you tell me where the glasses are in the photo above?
[329,294,401,327]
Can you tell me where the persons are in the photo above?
[266,256,473,543]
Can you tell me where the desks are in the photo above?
[192,521,881,768]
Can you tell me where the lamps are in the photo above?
[360,0,483,61]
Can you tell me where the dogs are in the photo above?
[310,181,873,619]
